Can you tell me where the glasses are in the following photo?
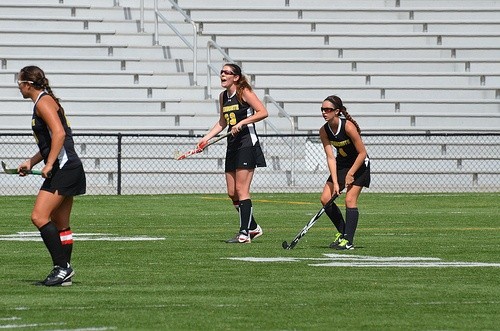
[321,107,336,113]
[221,70,236,76]
[17,81,33,86]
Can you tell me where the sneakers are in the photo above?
[43,262,76,286]
[226,233,251,244]
[335,239,355,250]
[249,224,263,239]
[329,232,344,247]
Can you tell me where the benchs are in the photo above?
[0,0,500,192]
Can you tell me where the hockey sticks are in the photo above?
[1,161,52,177]
[177,127,242,160]
[281,183,345,250]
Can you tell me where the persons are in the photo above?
[196,63,269,244]
[319,95,371,251]
[17,66,86,286]
